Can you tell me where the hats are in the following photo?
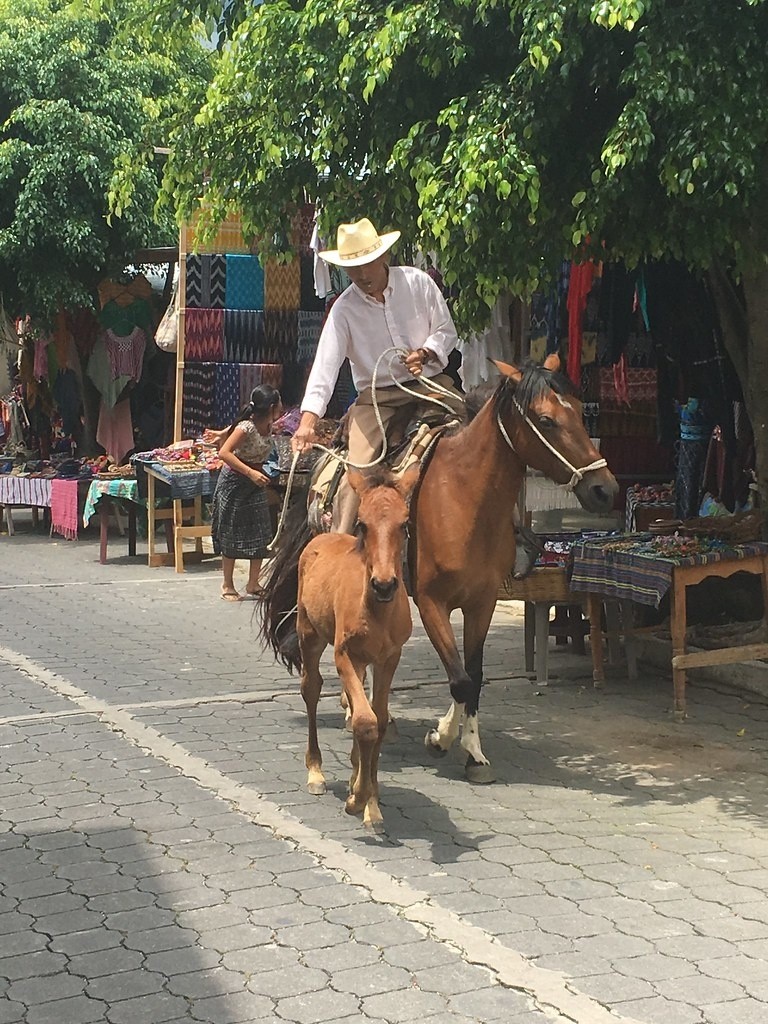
[0,456,89,481]
[318,218,401,268]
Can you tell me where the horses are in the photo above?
[254,351,621,836]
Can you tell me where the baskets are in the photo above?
[98,472,121,479]
[121,469,137,480]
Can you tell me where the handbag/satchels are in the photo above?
[154,275,177,353]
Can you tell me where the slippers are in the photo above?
[220,592,241,601]
[248,589,268,597]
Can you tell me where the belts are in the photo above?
[376,379,418,391]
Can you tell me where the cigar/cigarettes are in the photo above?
[368,282,372,287]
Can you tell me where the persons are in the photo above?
[203,383,282,601]
[290,218,469,536]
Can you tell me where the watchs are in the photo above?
[417,348,429,365]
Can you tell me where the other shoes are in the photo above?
[513,527,542,580]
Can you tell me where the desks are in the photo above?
[0,448,222,571]
[573,536,768,722]
